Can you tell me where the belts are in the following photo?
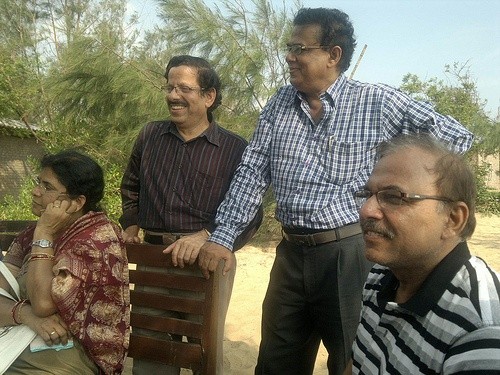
[281,222,362,246]
[143,232,184,245]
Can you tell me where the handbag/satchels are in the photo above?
[0,262,38,375]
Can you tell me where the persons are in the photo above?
[340,134,500,375]
[119,54,264,375]
[199,8,476,375]
[0,152,130,375]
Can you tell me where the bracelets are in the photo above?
[11,300,24,325]
[18,299,30,323]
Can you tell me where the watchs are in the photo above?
[31,239,54,248]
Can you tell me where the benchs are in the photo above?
[1,218,238,373]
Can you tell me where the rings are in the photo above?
[50,331,56,336]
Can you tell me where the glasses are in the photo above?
[160,85,205,96]
[283,45,331,55]
[33,175,63,195]
[353,187,453,208]
